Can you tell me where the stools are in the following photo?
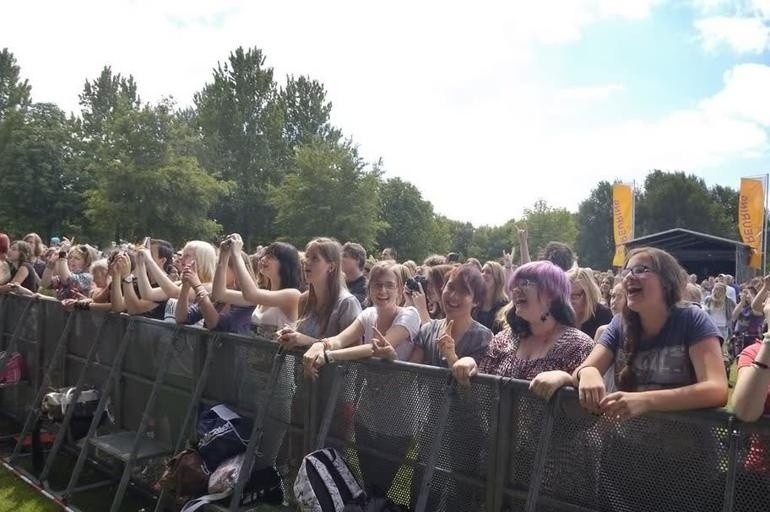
[0,350,294,512]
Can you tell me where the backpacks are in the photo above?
[293,445,410,512]
[159,401,285,507]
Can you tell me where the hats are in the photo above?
[508,277,537,292]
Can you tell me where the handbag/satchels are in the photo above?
[36,382,113,441]
[0,351,24,385]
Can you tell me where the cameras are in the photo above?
[405,275,426,293]
[220,235,231,250]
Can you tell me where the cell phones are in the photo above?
[143,237,151,248]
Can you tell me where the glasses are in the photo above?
[569,290,585,300]
[620,264,656,279]
